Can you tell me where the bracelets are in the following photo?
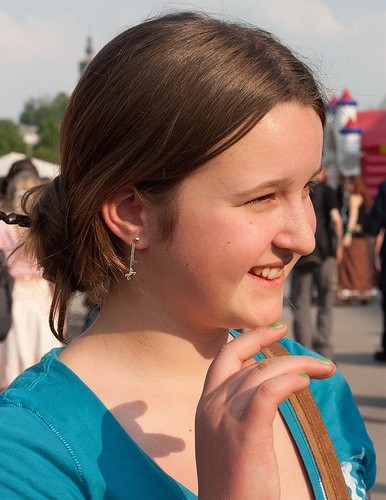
[344,228,353,235]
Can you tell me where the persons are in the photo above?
[336,175,378,305]
[367,178,386,365]
[291,164,344,366]
[1,161,69,392]
[0,13,379,500]
